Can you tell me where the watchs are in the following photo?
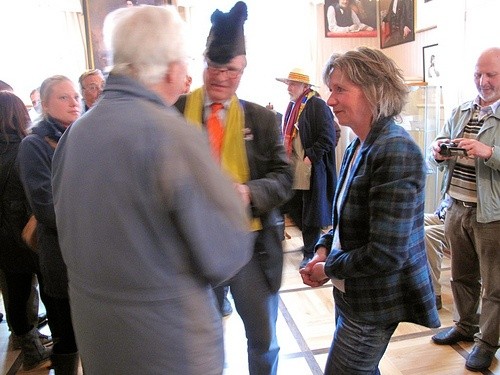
[487,147,494,160]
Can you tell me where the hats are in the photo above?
[276,71,320,90]
[204,1,248,63]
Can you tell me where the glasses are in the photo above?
[207,64,242,79]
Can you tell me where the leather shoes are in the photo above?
[431,327,475,345]
[465,345,494,372]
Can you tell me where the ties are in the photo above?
[206,102,226,168]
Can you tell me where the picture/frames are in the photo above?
[324,0,377,37]
[415,0,438,33]
[423,44,442,86]
[379,0,415,50]
[82,0,173,76]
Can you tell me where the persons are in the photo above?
[0,44,500,375]
[174,2,290,375]
[52,5,252,375]
[327,0,413,47]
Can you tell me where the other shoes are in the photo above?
[23,345,53,370]
[300,257,311,269]
[223,295,233,316]
[15,333,53,348]
[38,312,47,327]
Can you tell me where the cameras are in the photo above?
[439,141,467,158]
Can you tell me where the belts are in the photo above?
[456,200,477,208]
